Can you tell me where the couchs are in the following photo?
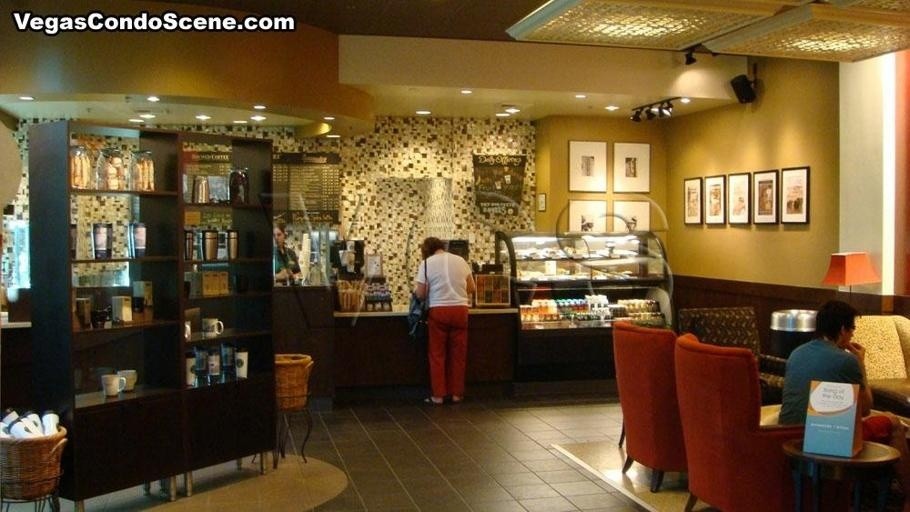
[609,318,689,496]
[836,310,910,415]
[672,331,808,512]
[677,305,790,400]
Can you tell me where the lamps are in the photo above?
[683,41,702,64]
[821,250,882,308]
[632,95,682,121]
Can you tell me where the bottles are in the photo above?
[521,297,664,328]
[310,262,322,284]
[190,174,210,202]
[228,166,250,205]
[71,143,154,190]
[346,252,355,272]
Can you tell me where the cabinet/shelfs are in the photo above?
[27,119,274,511]
[494,229,672,402]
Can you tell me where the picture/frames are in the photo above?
[704,175,726,226]
[780,166,810,225]
[567,140,608,196]
[682,176,703,225]
[611,141,651,194]
[614,199,651,232]
[537,192,546,212]
[728,172,751,226]
[752,170,780,225]
[568,199,607,233]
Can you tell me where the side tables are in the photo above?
[782,435,900,511]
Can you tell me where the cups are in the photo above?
[93,223,113,260]
[202,318,224,338]
[235,274,249,293]
[184,354,195,385]
[545,260,557,276]
[185,229,239,262]
[183,281,190,299]
[0,311,9,325]
[503,175,511,185]
[207,355,220,377]
[134,223,147,256]
[185,321,192,342]
[101,369,138,398]
[495,180,501,189]
[90,310,107,329]
[0,407,60,439]
[346,241,355,253]
[300,233,312,280]
[233,350,249,378]
[337,289,359,311]
[131,297,148,312]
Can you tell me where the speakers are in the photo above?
[730,75,756,104]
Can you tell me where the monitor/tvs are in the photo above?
[332,238,365,273]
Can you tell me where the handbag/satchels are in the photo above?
[408,293,428,341]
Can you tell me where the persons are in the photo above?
[690,187,696,215]
[416,237,475,405]
[763,185,772,213]
[786,186,802,212]
[779,299,904,452]
[732,196,745,216]
[711,192,720,215]
[274,222,301,286]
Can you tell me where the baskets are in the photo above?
[0,426,69,499]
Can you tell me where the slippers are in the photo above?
[421,395,442,406]
[451,392,464,405]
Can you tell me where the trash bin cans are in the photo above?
[766,309,820,361]
